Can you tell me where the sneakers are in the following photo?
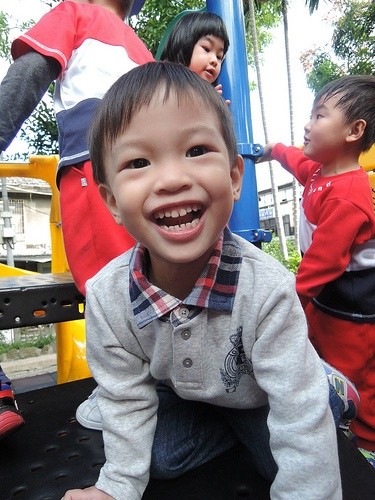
[320,357,361,420]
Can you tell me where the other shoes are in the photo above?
[76,386,107,430]
[0,405,26,439]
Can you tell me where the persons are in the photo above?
[60,61,359,500]
[0,364,25,436]
[297,73,374,472]
[166,12,232,106]
[0,0,161,298]
